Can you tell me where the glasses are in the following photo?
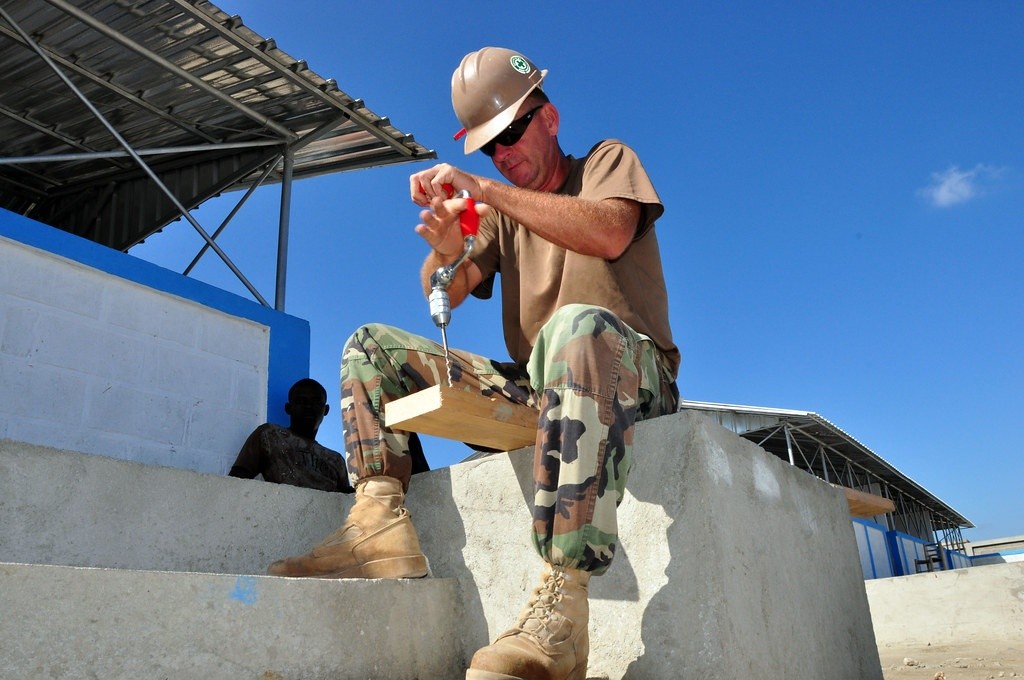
[479,105,543,156]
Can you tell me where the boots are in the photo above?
[266,476,429,579]
[466,563,591,680]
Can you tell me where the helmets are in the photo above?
[451,46,549,155]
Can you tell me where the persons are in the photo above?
[228,377,355,494]
[267,46,681,680]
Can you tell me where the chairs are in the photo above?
[914,542,946,573]
